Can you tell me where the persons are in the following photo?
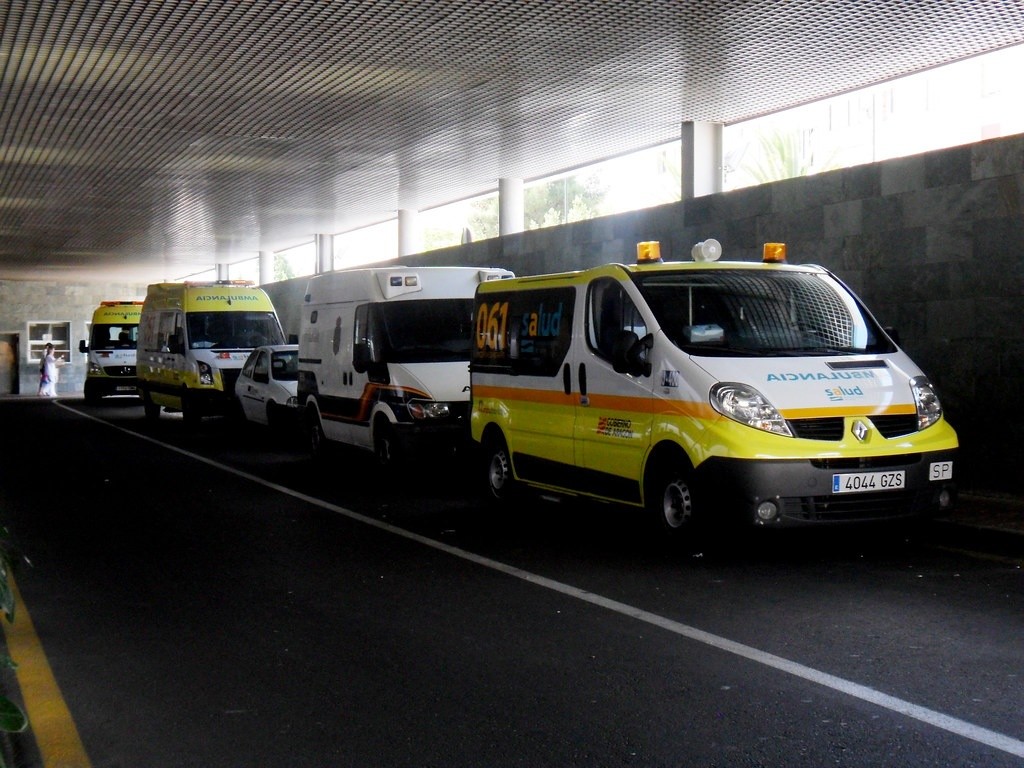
[42,345,60,397]
[38,342,55,395]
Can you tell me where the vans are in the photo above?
[136,282,288,434]
[294,264,517,483]
[79,301,160,408]
[462,237,960,567]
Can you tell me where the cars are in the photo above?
[232,345,315,435]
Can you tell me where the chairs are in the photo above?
[725,296,804,351]
[267,358,289,384]
[608,331,645,376]
[115,332,136,349]
